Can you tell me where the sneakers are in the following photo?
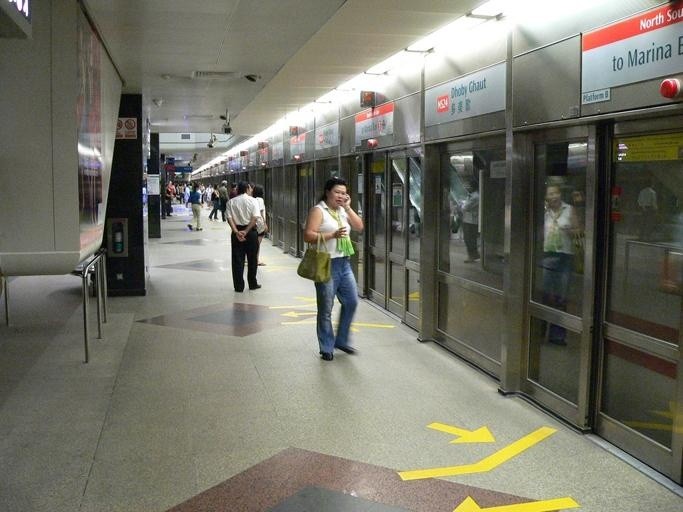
[196,227,203,231]
[188,224,193,231]
[334,343,354,354]
[322,351,333,361]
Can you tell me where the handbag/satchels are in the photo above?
[185,202,193,208]
[297,230,332,283]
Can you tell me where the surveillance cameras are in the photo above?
[222,124,232,135]
[188,161,195,167]
[207,139,218,148]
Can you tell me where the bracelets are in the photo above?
[342,206,351,213]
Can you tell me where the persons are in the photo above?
[539,179,582,348]
[251,184,267,267]
[302,175,365,361]
[223,179,261,292]
[159,173,238,233]
[456,177,480,265]
[633,177,660,242]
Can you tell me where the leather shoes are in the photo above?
[248,284,261,290]
[234,286,243,293]
